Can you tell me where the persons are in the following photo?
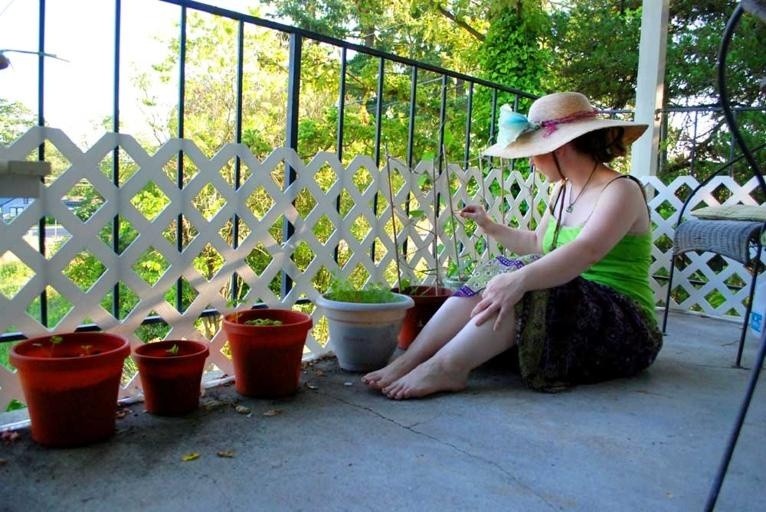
[362,91,663,400]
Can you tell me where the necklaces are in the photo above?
[564,163,599,213]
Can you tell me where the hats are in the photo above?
[480,90,652,160]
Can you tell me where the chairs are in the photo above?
[658,139,766,374]
[707,0,766,510]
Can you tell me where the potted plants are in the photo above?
[6,332,133,449]
[220,307,316,400]
[392,279,451,354]
[315,280,415,376]
[129,339,209,418]
